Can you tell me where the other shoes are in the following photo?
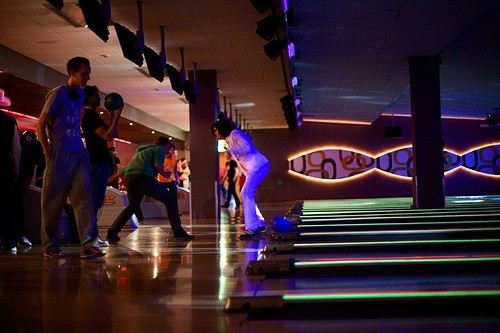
[106,233,120,240]
[43,248,73,257]
[240,229,260,238]
[98,238,109,246]
[80,246,106,257]
[259,225,266,231]
[174,231,194,240]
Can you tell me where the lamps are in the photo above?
[249,0,299,131]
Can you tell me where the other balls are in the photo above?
[105,92,124,113]
[271,215,291,234]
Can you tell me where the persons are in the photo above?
[81,85,125,247]
[0,87,79,253]
[36,56,107,258]
[103,143,191,217]
[213,116,270,239]
[106,137,195,240]
[216,151,246,211]
